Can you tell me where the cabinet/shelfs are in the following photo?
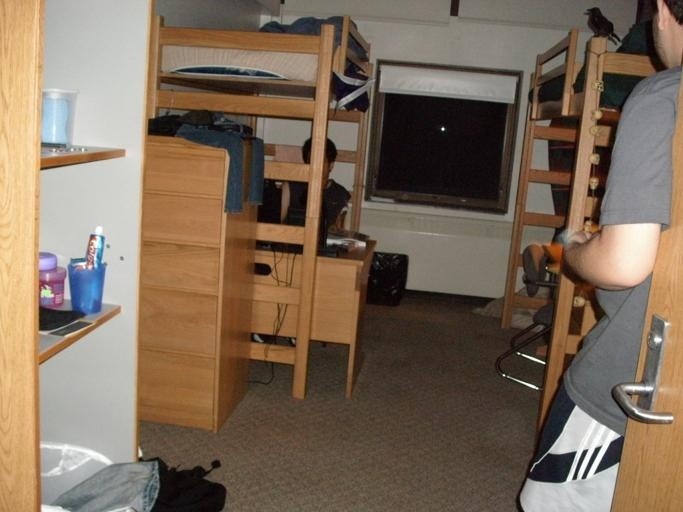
[135,134,262,435]
[37,141,125,366]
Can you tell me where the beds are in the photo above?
[150,13,377,402]
[503,25,668,438]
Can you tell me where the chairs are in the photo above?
[496,245,578,397]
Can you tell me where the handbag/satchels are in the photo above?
[139,457,227,512]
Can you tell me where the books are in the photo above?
[50,319,96,341]
[38,304,87,335]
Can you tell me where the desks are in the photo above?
[255,229,385,398]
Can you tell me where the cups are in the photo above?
[67,258,107,314]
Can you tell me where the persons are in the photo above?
[510,1,683,512]
[248,134,351,348]
